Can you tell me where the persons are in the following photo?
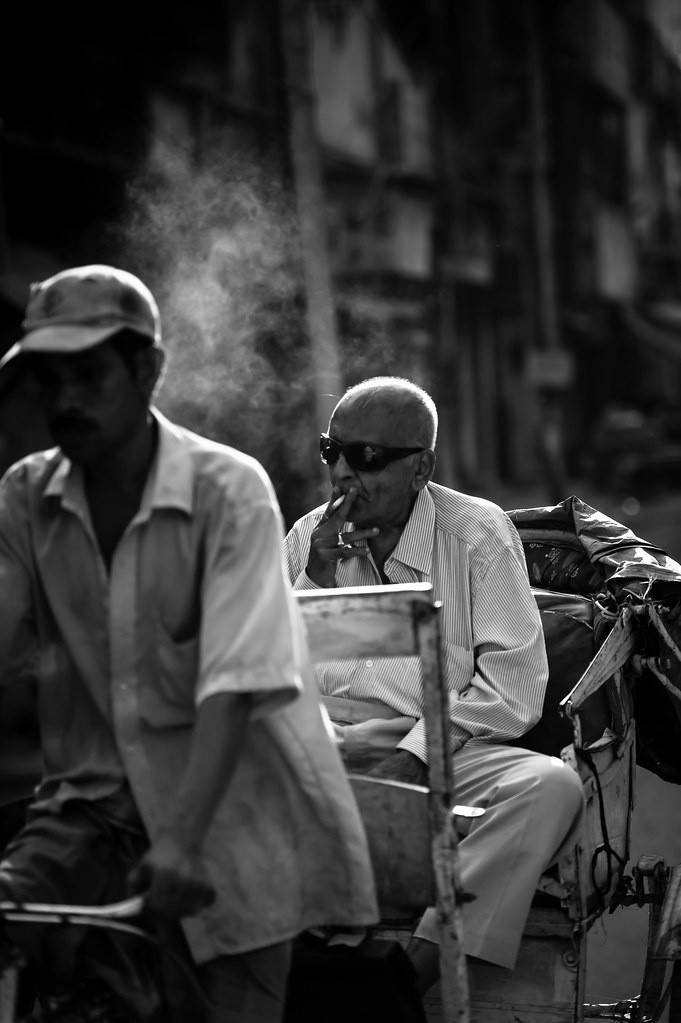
[279,374,588,1023]
[0,266,378,1023]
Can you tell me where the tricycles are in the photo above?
[0,493,680,1023]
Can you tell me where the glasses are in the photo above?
[319,432,425,472]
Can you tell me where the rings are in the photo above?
[322,514,330,521]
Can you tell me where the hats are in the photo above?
[0,264,162,368]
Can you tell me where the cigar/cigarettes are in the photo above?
[332,492,347,509]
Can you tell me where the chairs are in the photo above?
[498,532,637,818]
[274,582,468,1023]
